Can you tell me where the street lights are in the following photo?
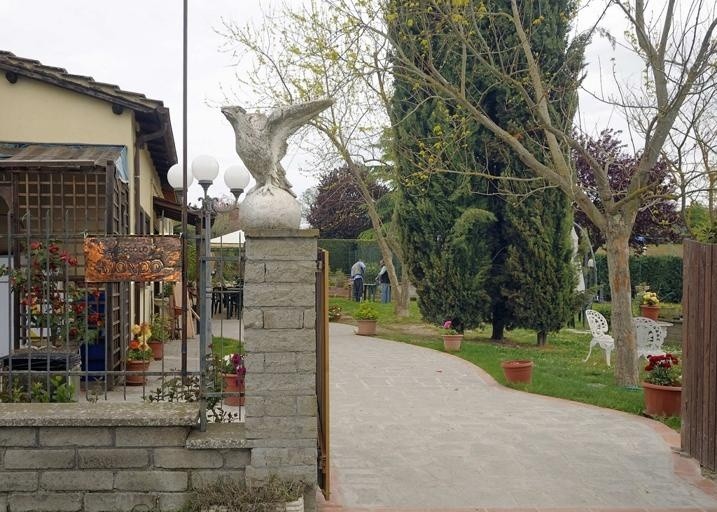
[164,148,250,432]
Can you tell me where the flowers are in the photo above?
[644,352,678,386]
[443,320,456,335]
[126,323,152,360]
[221,352,246,386]
[642,291,659,305]
[0,237,104,343]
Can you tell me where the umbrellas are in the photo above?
[569,227,586,294]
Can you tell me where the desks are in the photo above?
[362,284,376,302]
[656,321,675,339]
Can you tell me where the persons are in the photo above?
[375,260,390,303]
[350,258,366,303]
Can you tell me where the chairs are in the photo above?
[633,316,669,362]
[584,309,615,366]
[208,280,244,320]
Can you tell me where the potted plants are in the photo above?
[146,312,174,361]
[351,299,378,336]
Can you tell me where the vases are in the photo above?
[219,373,246,406]
[441,335,464,352]
[640,380,682,417]
[22,344,81,355]
[126,360,149,386]
[639,305,661,322]
[501,359,533,384]
